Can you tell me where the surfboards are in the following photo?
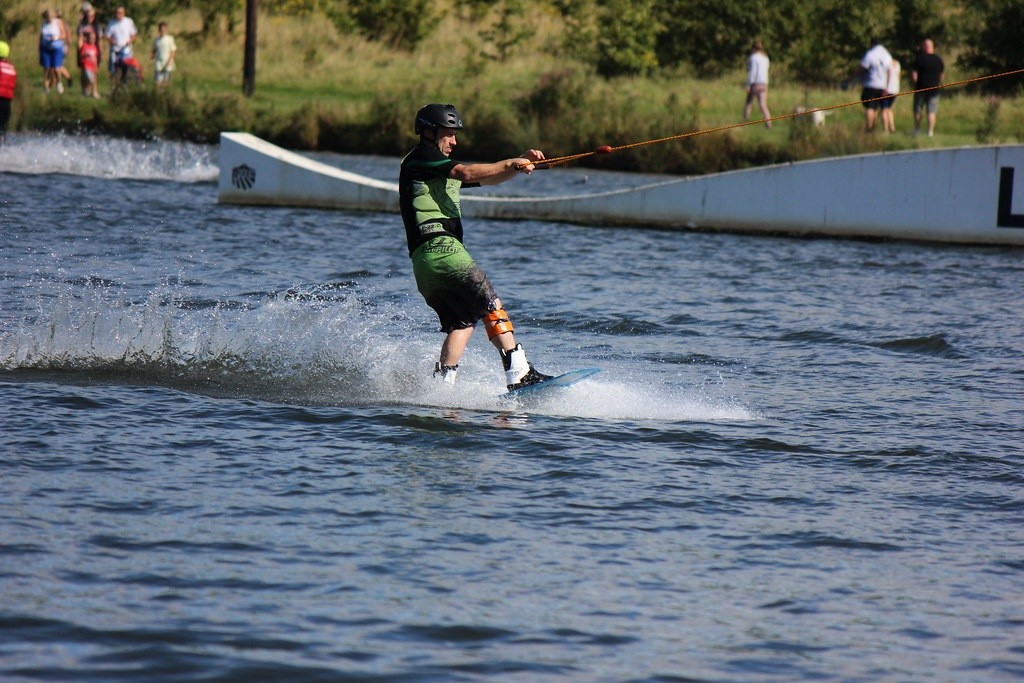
[487,368,601,400]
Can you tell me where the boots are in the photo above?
[433,362,459,383]
[499,343,554,392]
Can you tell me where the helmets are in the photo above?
[415,104,463,135]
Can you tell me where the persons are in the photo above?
[911,38,944,136]
[881,44,901,134]
[76,2,101,99]
[150,20,177,86]
[847,37,893,131]
[400,105,555,392]
[105,6,138,93]
[0,40,16,132]
[40,9,73,94]
[743,41,773,129]
